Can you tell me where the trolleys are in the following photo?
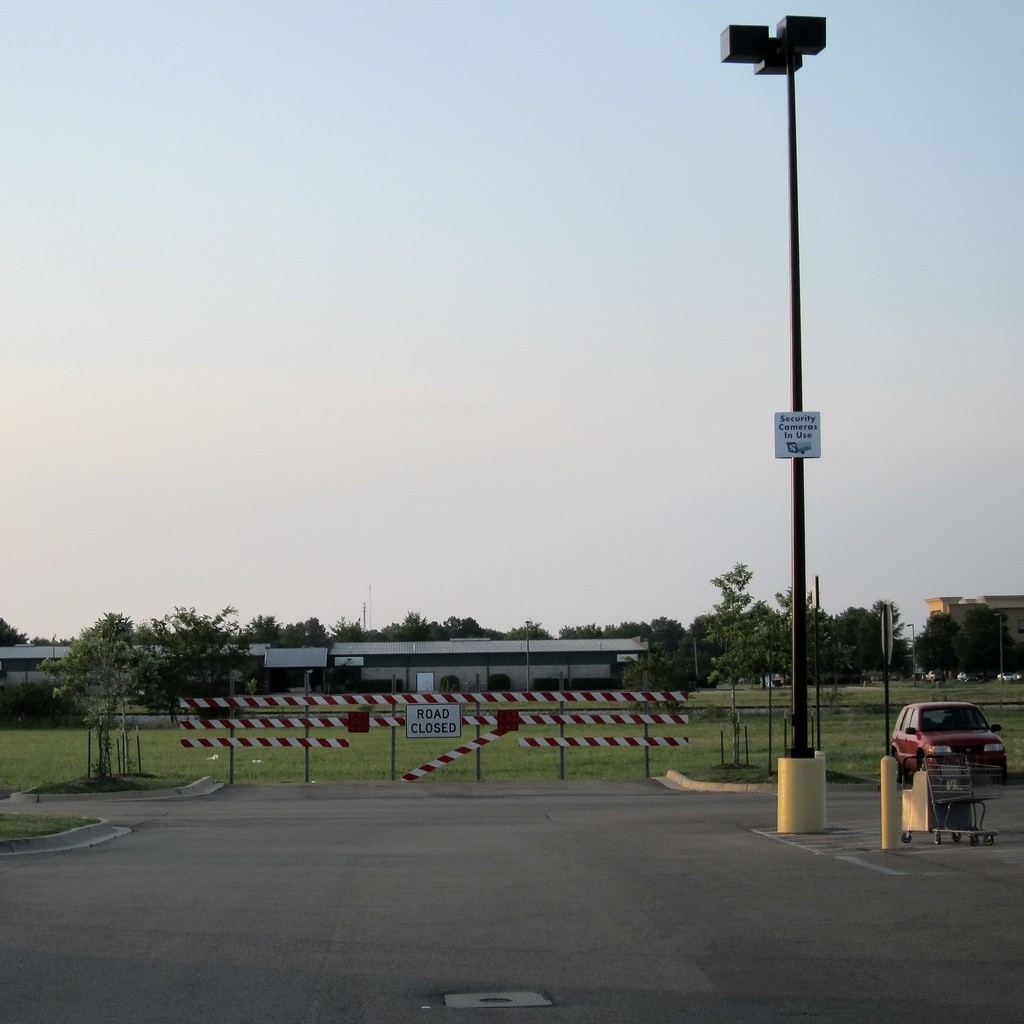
[921,748,1004,846]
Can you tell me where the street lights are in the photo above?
[721,16,828,835]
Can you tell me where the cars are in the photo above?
[890,699,1008,787]
